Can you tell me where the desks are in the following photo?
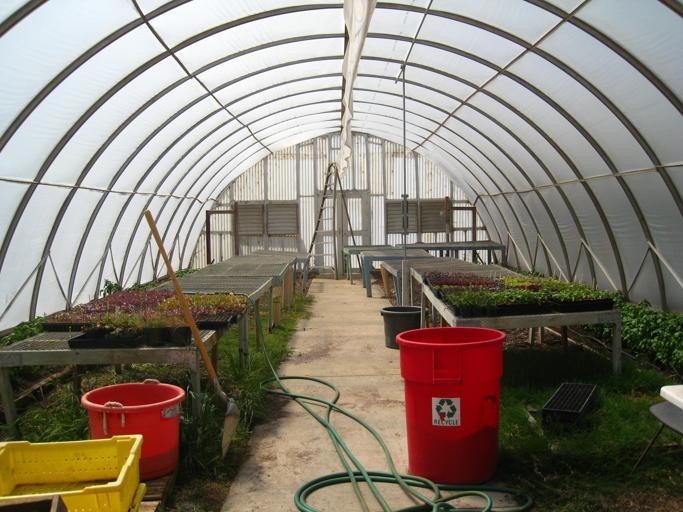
[420,276,621,378]
[343,240,522,328]
[148,250,311,369]
[0,290,244,430]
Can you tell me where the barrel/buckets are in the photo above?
[380,306,428,349]
[81,379,185,481]
[396,327,506,483]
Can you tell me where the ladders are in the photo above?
[302,163,362,280]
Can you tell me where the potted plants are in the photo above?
[440,286,553,318]
[41,289,174,331]
[553,288,614,313]
[500,273,584,292]
[68,310,148,347]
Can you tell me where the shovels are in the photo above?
[144,210,240,460]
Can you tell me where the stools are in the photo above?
[628,403,683,478]
